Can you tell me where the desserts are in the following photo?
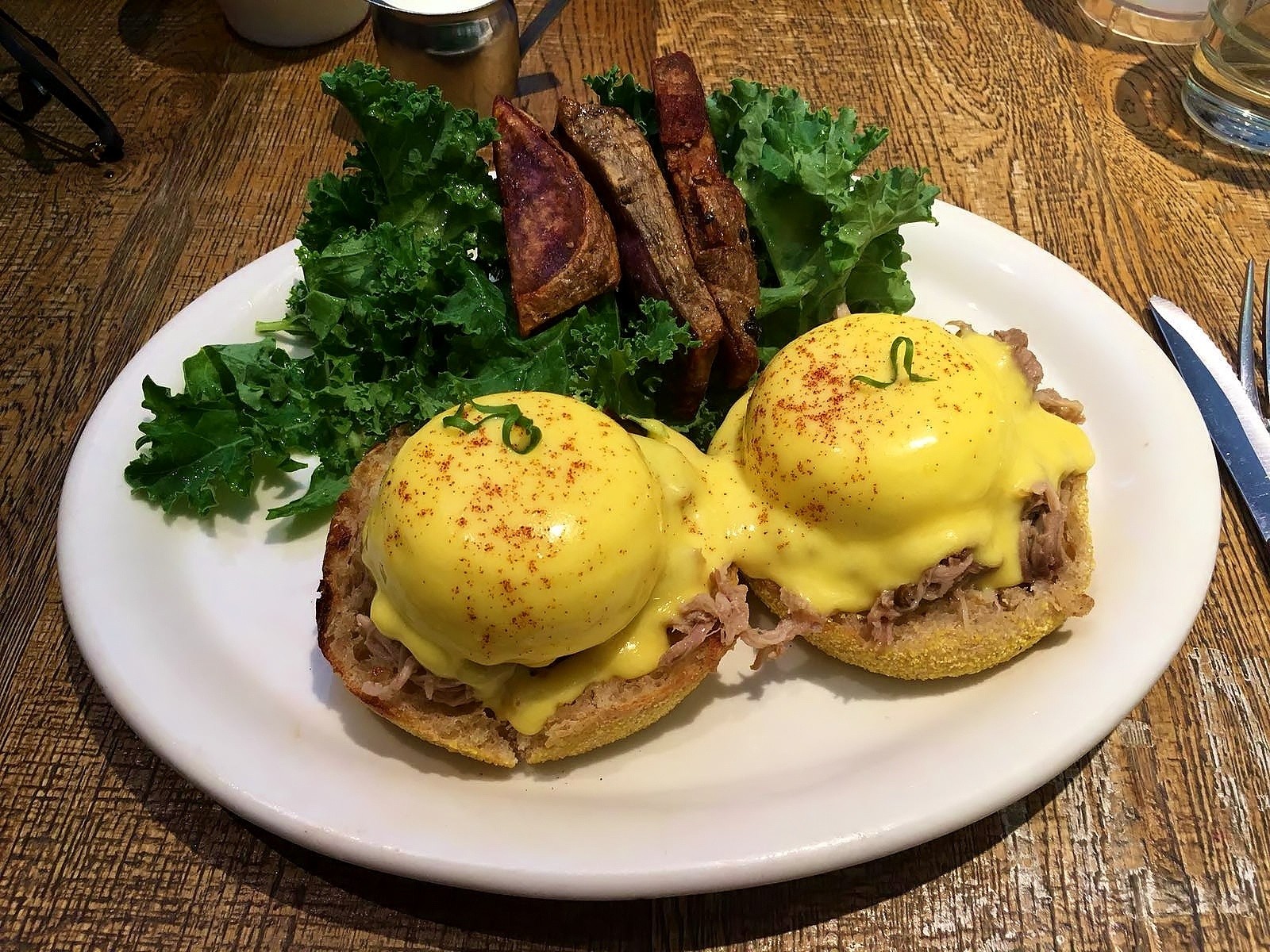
[703,310,1093,677]
[313,391,754,771]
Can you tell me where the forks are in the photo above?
[1239,257,1270,434]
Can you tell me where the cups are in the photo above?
[362,0,522,143]
[1076,0,1213,46]
[1180,0,1270,156]
[220,0,373,56]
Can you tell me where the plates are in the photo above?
[57,170,1223,899]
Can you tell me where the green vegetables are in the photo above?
[122,62,940,521]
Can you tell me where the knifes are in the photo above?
[1143,293,1270,565]
